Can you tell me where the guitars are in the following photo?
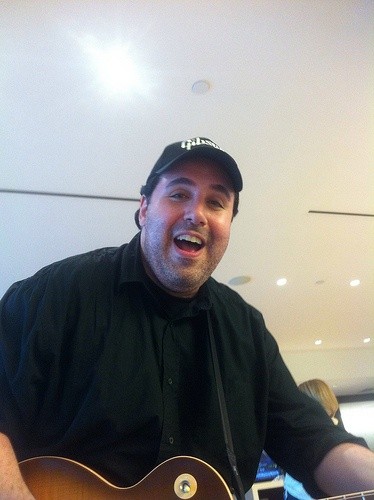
[18,455,374,500]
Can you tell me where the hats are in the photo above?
[147,136,243,193]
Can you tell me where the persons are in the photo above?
[282,379,340,500]
[0,137,374,500]
